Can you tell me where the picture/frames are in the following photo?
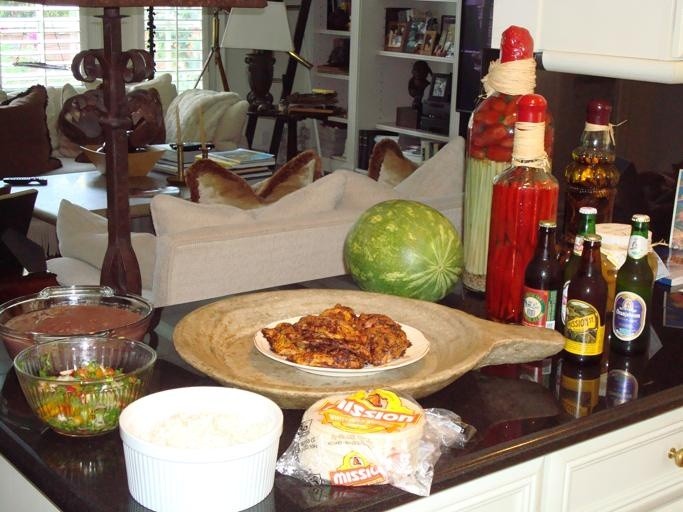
[384,7,456,58]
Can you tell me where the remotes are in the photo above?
[3,177,47,185]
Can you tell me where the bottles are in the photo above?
[562,206,598,280]
[562,236,604,373]
[485,93,558,321]
[564,100,618,227]
[612,215,653,353]
[519,218,557,370]
[464,25,555,298]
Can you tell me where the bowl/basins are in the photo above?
[1,285,153,359]
[120,386,283,511]
[13,337,157,438]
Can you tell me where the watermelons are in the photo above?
[342,199,464,302]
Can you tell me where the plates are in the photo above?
[254,316,431,377]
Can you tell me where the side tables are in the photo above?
[247,104,338,170]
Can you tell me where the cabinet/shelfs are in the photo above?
[384,403,682,512]
[202,0,461,185]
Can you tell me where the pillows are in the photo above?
[368,140,419,187]
[250,150,324,205]
[1,85,62,181]
[185,159,265,210]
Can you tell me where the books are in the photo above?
[297,122,347,157]
[358,130,447,171]
[150,144,275,180]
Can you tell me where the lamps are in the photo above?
[220,1,294,112]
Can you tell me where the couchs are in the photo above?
[0,74,250,176]
[47,137,464,310]
